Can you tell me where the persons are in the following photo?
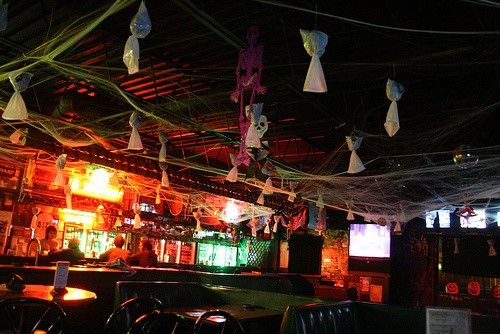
[48,239,85,265]
[99,236,130,267]
[39,226,58,255]
[130,239,158,268]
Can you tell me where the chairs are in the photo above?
[0,297,68,334]
[105,297,243,334]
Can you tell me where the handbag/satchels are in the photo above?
[110,257,133,271]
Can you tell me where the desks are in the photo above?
[0,284,98,310]
[158,304,281,328]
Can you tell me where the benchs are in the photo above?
[278,300,363,334]
[113,281,201,334]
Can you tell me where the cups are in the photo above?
[243,304,251,311]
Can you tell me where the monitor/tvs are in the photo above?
[347,222,393,261]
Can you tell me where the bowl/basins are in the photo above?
[5,274,25,293]
